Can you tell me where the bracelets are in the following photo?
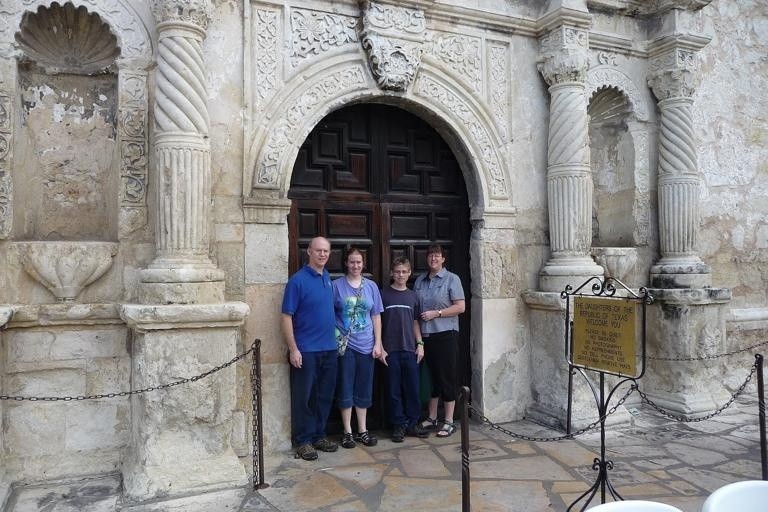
[416,340,425,345]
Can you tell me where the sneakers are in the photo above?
[406,424,430,438]
[294,443,318,461]
[313,437,339,452]
[391,424,405,442]
[341,432,356,448]
[355,430,378,446]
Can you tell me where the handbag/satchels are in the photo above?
[336,335,349,358]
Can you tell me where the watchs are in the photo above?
[438,310,441,318]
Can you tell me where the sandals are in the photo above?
[420,416,438,430]
[436,421,456,438]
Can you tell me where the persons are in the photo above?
[281,236,339,460]
[379,255,429,443]
[332,247,385,449]
[413,244,466,437]
